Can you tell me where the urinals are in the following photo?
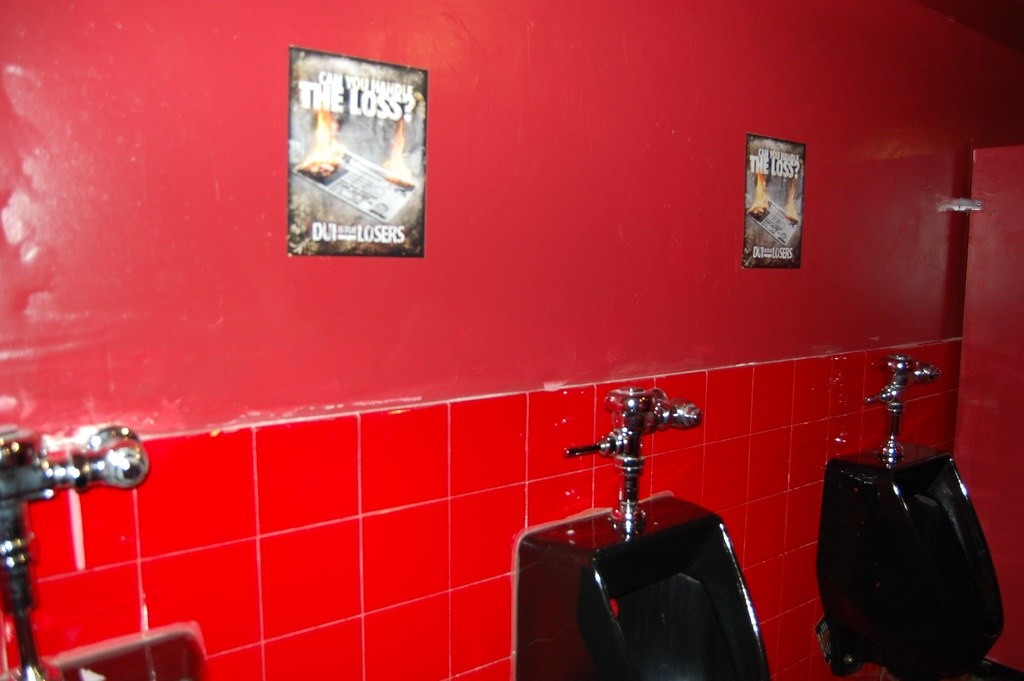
[814,434,1005,681]
[514,490,773,681]
